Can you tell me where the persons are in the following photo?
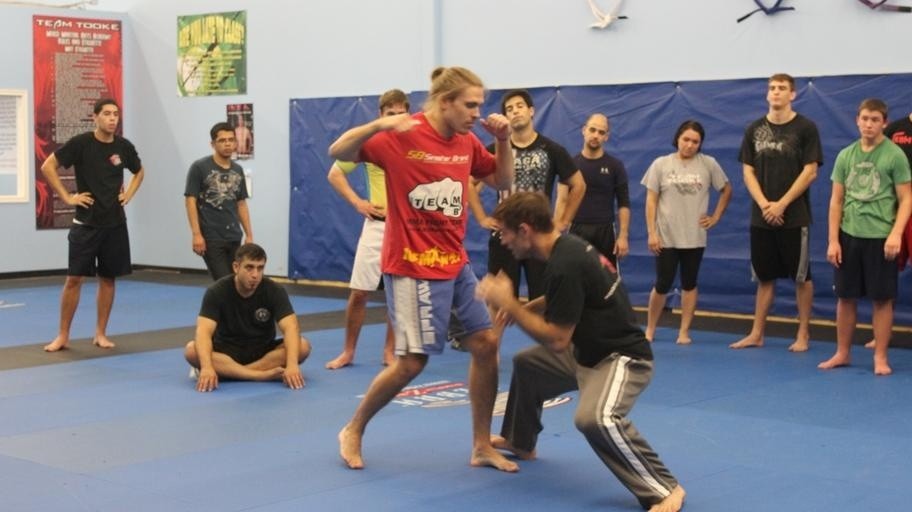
[729,73,825,352]
[40,98,144,352]
[474,192,687,512]
[468,89,587,365]
[184,243,310,392]
[640,121,733,345]
[329,67,520,472]
[864,112,912,350]
[324,89,410,370]
[817,98,911,376]
[554,113,631,274]
[184,122,253,282]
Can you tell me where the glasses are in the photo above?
[213,138,236,142]
